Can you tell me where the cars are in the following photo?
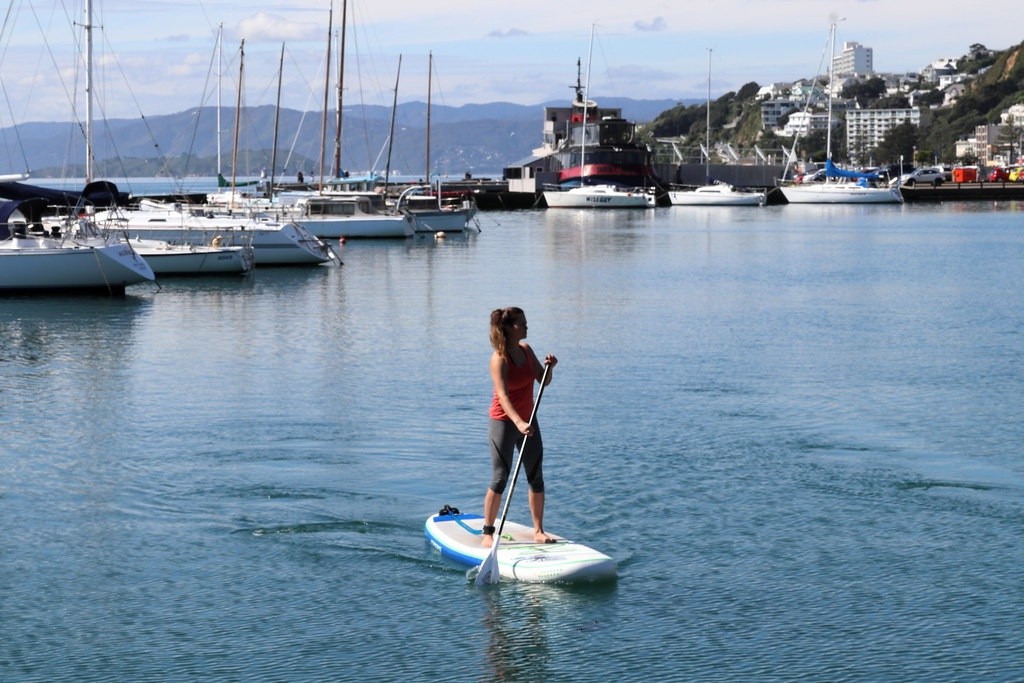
[988,165,1024,184]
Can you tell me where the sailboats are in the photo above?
[542,22,659,209]
[668,47,768,206]
[0,1,470,300]
[773,22,904,204]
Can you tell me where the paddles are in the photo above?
[469,359,554,586]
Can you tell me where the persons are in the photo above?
[481,307,558,547]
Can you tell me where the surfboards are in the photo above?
[424,509,617,590]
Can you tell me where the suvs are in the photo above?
[877,163,915,182]
[901,167,945,187]
